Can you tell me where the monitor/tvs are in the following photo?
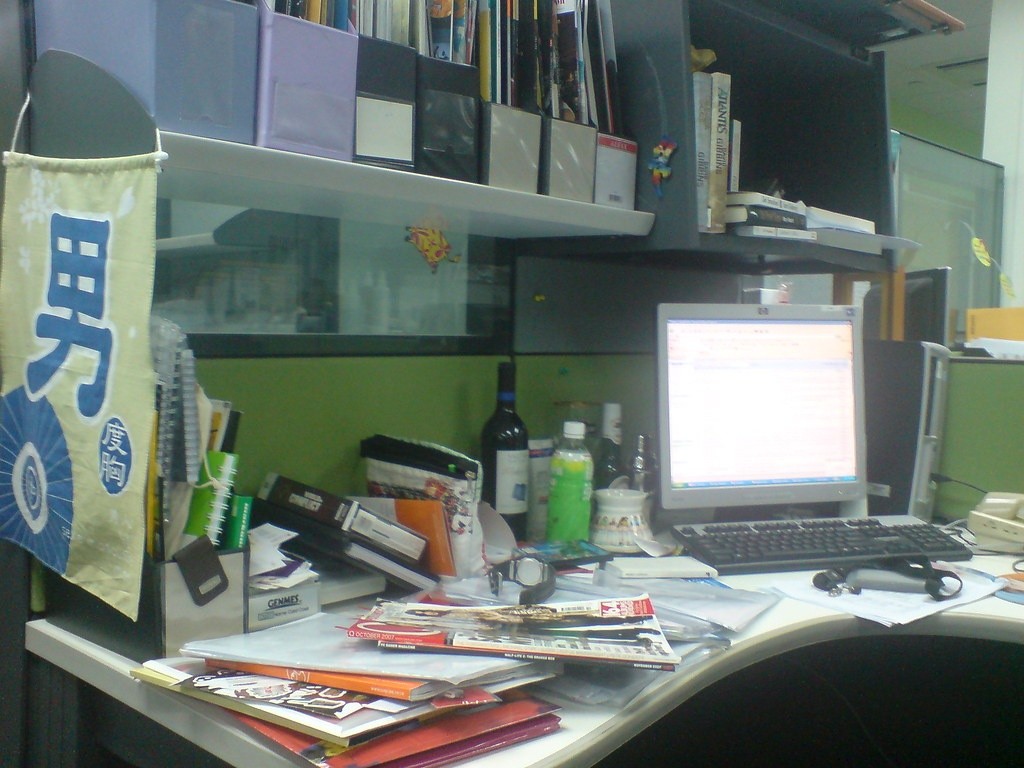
[654,301,869,520]
[867,265,953,348]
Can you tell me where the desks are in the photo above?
[24,522,1024,768]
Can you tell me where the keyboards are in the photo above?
[670,515,973,575]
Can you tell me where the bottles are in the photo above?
[590,401,634,491]
[481,361,529,548]
[545,421,594,543]
[627,431,659,535]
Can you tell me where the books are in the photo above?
[275,0,882,258]
[128,315,782,768]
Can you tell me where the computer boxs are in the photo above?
[862,340,948,524]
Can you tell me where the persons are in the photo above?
[405,606,661,647]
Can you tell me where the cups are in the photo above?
[590,489,655,555]
